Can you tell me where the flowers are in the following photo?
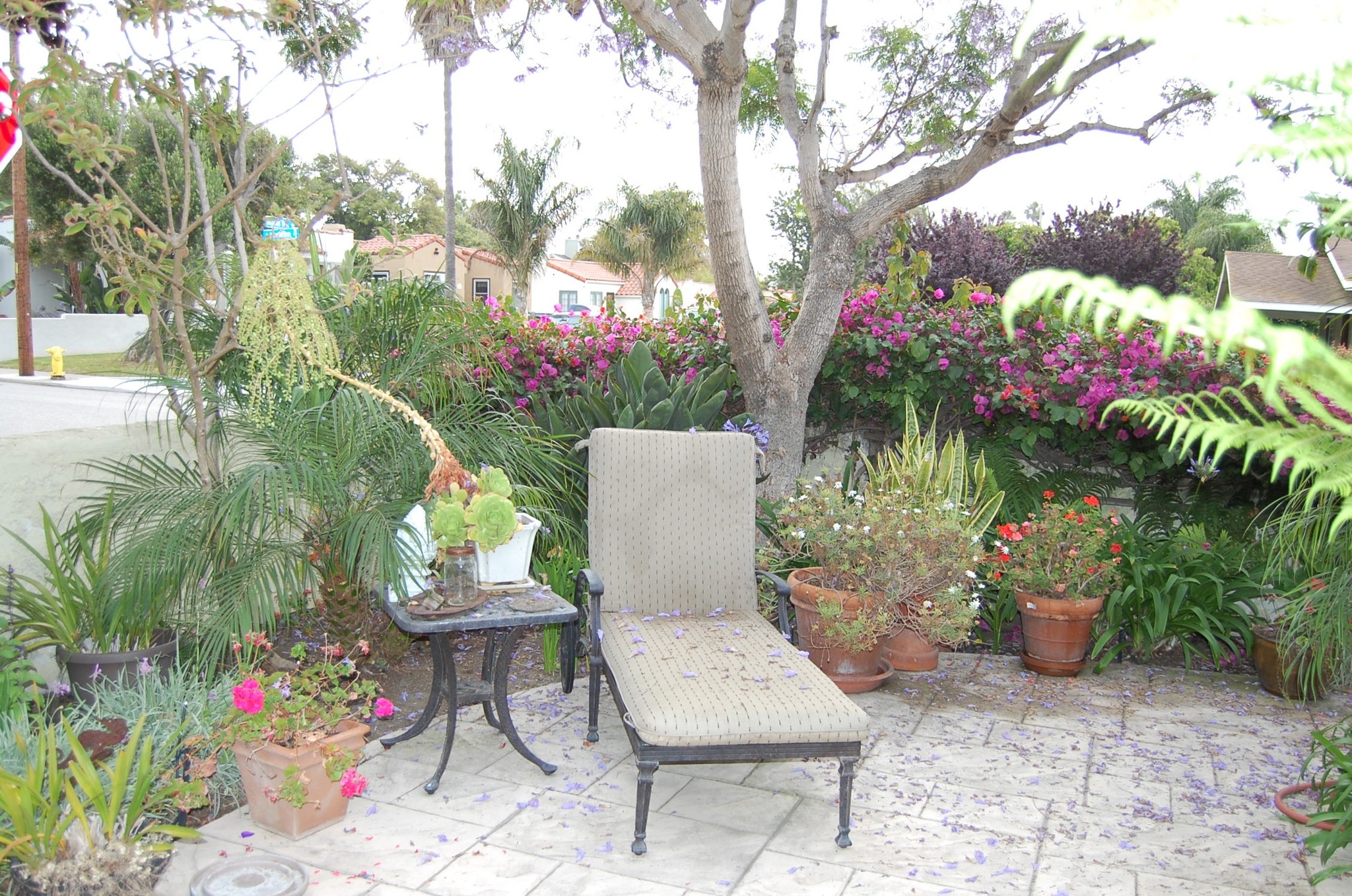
[772,470,981,596]
[1290,578,1333,639]
[224,632,395,809]
[986,489,1122,596]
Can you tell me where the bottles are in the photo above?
[444,547,478,606]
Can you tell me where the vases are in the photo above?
[785,565,904,685]
[228,727,378,843]
[1009,583,1112,676]
[1254,622,1342,701]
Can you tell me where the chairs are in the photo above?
[572,425,874,854]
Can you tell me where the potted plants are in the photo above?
[0,712,205,896]
[856,394,1005,672]
[427,469,541,583]
[0,518,177,718]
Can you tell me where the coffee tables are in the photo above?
[374,579,580,795]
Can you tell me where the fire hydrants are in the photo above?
[47,346,67,379]
